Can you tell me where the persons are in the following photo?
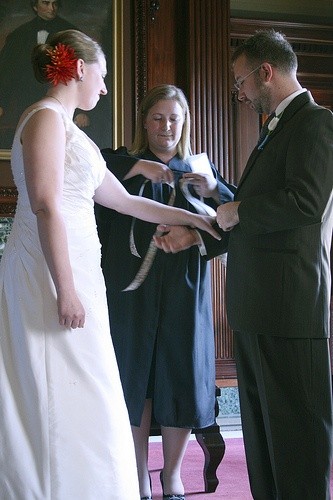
[0,28,222,500]
[93,84,237,500]
[152,28,333,500]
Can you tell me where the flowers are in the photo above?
[45,43,77,86]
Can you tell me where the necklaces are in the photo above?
[46,96,67,112]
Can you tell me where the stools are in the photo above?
[149,386,225,493]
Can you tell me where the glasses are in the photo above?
[234,63,262,91]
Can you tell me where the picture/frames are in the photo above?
[0,0,124,161]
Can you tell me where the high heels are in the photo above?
[159,469,185,500]
[141,473,152,500]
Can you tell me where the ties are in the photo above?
[233,112,276,187]
